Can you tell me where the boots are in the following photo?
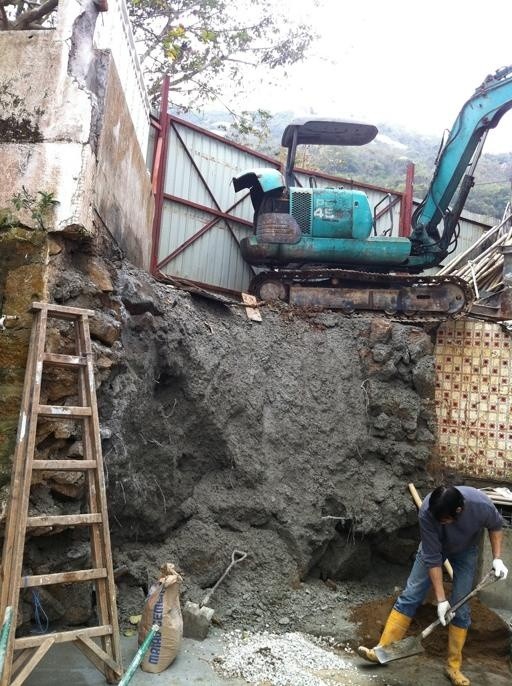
[359,609,410,665]
[444,625,472,686]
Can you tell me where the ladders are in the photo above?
[0,299,123,686]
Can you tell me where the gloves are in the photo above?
[491,558,507,580]
[438,600,456,626]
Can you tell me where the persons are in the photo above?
[355,484,508,686]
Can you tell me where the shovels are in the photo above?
[181,549,247,642]
[373,569,501,664]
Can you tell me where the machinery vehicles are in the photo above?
[232,64,511,324]
